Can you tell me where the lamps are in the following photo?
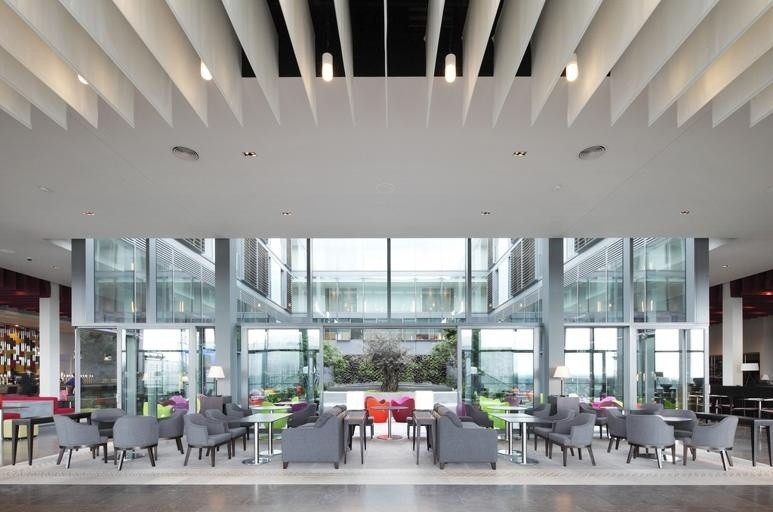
[207,366,226,396]
[740,362,759,386]
[552,366,571,397]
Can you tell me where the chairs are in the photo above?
[524,394,739,471]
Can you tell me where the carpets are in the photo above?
[0,441,773,486]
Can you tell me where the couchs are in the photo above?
[0,394,75,418]
[428,403,499,471]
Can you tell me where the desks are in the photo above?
[411,408,437,465]
[11,413,91,466]
[693,411,773,467]
[687,394,773,418]
[487,405,555,465]
